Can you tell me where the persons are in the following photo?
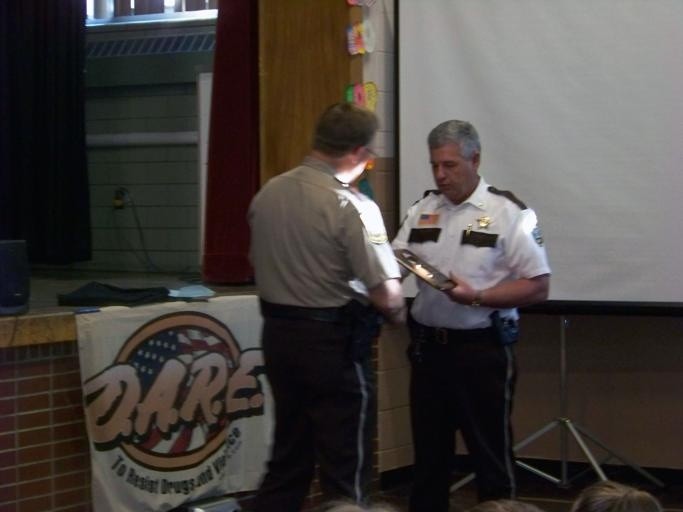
[245,101,409,512]
[468,499,545,512]
[389,119,553,512]
[570,480,663,512]
[312,499,398,511]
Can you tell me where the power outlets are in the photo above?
[112,189,128,204]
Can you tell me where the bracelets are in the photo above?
[471,290,482,308]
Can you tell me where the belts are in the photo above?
[417,326,489,343]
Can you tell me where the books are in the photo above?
[391,249,458,292]
